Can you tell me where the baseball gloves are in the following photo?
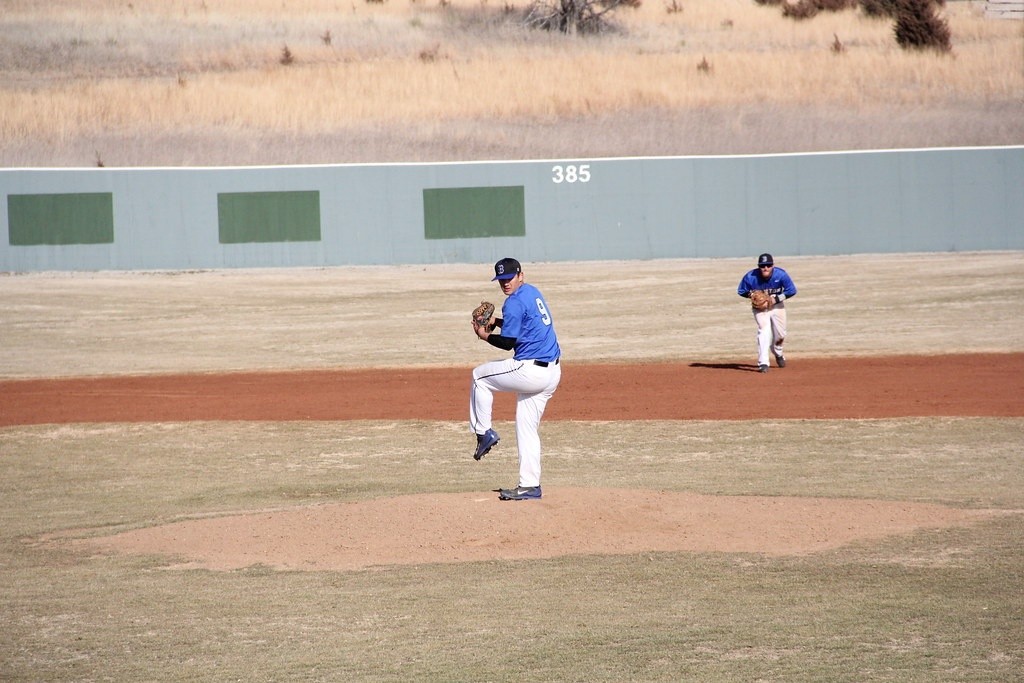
[471,302,497,334]
[751,290,772,310]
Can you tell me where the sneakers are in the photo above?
[760,364,770,373]
[776,356,785,367]
[500,484,542,500]
[473,428,500,462]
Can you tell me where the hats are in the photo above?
[758,254,773,266]
[491,258,521,282]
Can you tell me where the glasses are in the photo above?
[759,264,772,268]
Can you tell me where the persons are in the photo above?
[738,254,797,371]
[469,258,561,499]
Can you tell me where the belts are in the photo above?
[533,357,559,367]
